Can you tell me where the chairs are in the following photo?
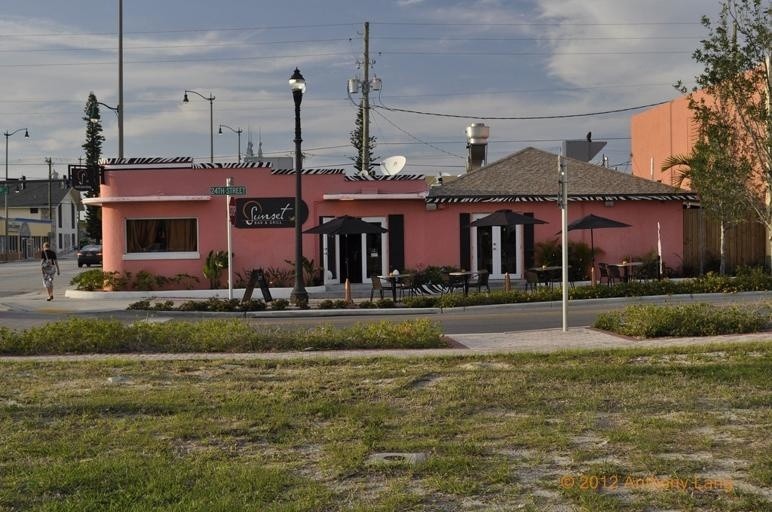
[524,265,575,290]
[370,272,425,302]
[599,262,649,287]
[440,271,490,296]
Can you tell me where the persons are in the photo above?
[38,241,60,301]
[387,264,400,284]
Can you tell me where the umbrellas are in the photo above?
[551,213,631,286]
[465,206,553,290]
[301,215,390,302]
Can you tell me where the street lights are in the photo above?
[183,90,242,162]
[86,103,123,157]
[3,128,29,261]
[289,69,307,291]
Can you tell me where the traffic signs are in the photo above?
[207,186,246,194]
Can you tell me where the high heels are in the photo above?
[47,295,53,301]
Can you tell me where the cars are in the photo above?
[77,245,102,267]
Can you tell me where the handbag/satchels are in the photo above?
[43,264,56,275]
